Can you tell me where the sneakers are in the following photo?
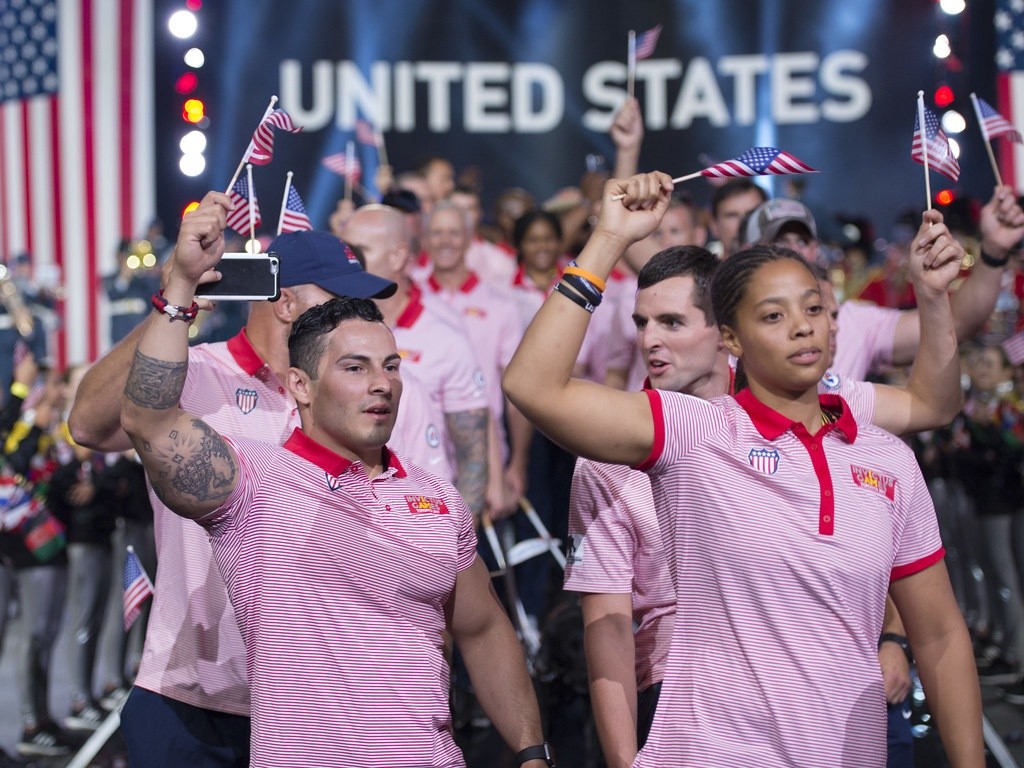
[20,721,69,755]
[96,682,129,710]
[963,616,1024,705]
[58,702,101,729]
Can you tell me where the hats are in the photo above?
[262,229,398,302]
[737,199,815,251]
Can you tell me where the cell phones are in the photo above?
[193,251,282,302]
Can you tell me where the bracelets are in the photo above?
[151,289,199,321]
[564,266,606,292]
[553,282,595,313]
[980,247,1009,267]
[562,274,601,307]
[568,259,602,301]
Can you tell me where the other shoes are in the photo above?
[471,700,490,728]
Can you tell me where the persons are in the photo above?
[0,98,1024,760]
[123,190,554,768]
[563,209,961,768]
[68,230,446,768]
[500,169,984,768]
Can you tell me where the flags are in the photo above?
[223,25,1024,366]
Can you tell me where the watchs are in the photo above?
[515,741,556,768]
[878,633,913,665]
